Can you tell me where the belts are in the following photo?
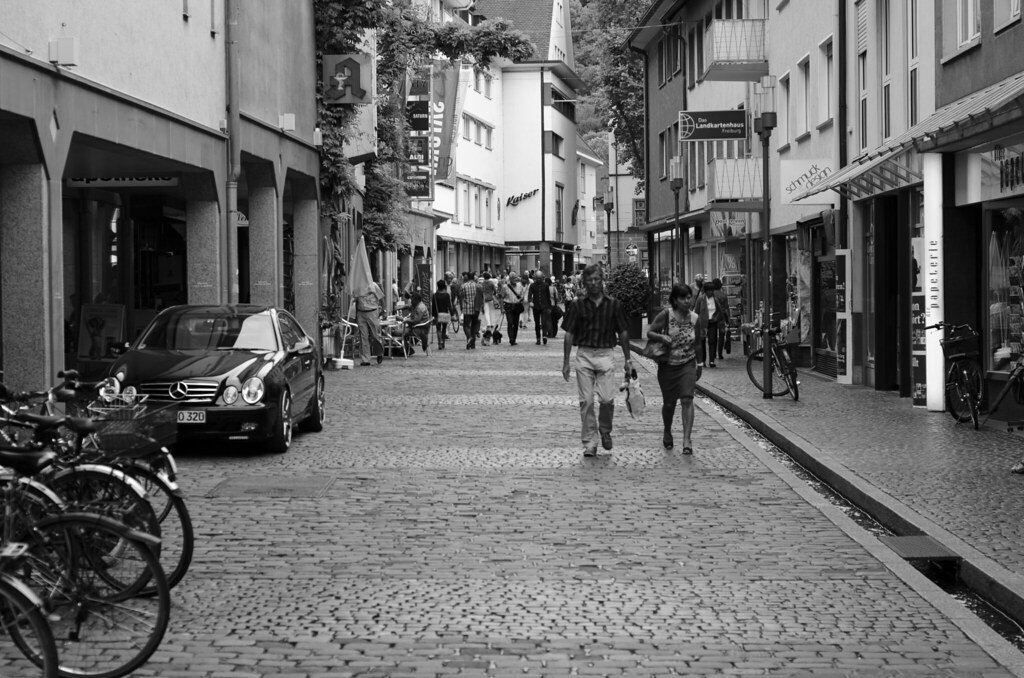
[358,309,377,313]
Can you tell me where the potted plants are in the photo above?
[605,260,652,339]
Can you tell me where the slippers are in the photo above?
[663,437,692,455]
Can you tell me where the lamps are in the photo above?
[313,131,322,146]
[279,113,296,131]
[49,35,79,66]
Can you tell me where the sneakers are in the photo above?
[584,432,612,457]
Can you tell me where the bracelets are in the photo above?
[625,360,632,363]
[697,362,704,366]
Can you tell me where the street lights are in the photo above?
[605,202,613,269]
[755,112,777,399]
[670,178,683,283]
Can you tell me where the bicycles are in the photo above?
[923,322,986,430]
[0,367,194,678]
[747,311,799,401]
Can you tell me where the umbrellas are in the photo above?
[340,235,376,358]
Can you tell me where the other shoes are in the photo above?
[466,337,475,348]
[536,337,547,345]
[361,356,383,366]
[510,339,517,345]
[709,362,717,367]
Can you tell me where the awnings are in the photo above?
[791,77,1024,202]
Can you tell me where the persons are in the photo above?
[648,283,705,454]
[689,273,732,368]
[357,282,385,365]
[562,265,634,456]
[85,315,106,360]
[392,269,587,355]
[68,292,108,348]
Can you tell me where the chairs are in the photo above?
[340,317,435,360]
[160,325,192,348]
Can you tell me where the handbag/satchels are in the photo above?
[620,370,645,419]
[724,329,731,354]
[518,303,524,313]
[438,312,450,323]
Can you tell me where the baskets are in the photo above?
[939,334,980,359]
[95,402,182,458]
[87,394,151,428]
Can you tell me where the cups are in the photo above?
[382,310,387,320]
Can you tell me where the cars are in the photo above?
[106,303,324,454]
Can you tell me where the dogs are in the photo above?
[492,323,503,345]
[480,324,494,347]
